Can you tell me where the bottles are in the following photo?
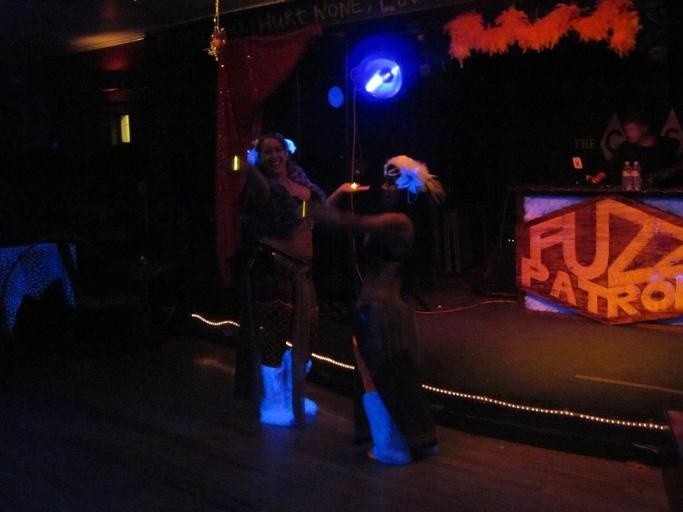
[632,161,643,192]
[621,161,632,191]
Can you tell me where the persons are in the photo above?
[591,115,683,188]
[236,133,370,427]
[326,156,439,465]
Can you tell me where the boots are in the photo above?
[257,347,319,428]
[361,390,440,467]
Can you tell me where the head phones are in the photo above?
[627,118,649,135]
